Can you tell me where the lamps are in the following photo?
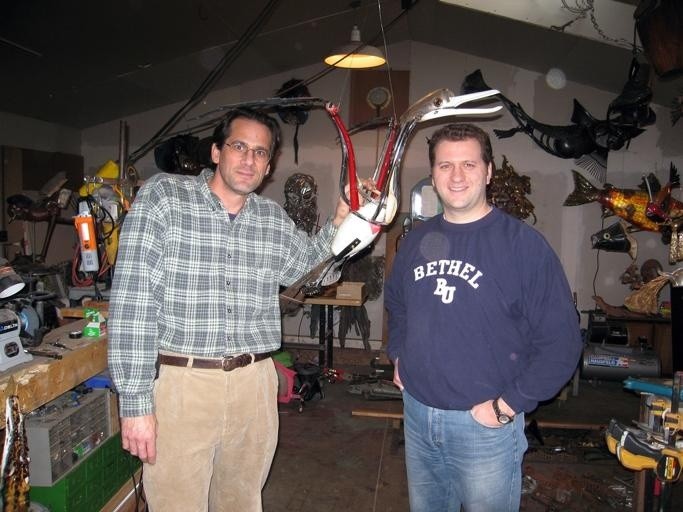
[321,4,387,70]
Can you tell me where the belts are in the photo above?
[157,352,270,371]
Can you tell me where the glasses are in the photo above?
[221,138,271,162]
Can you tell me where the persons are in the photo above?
[105,104,378,512]
[382,120,586,511]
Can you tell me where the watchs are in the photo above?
[492,397,513,425]
